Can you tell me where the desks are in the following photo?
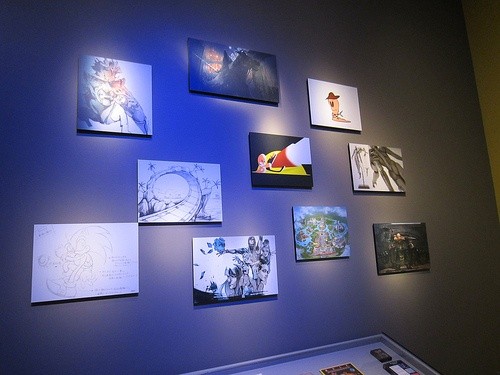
[178,332,440,375]
